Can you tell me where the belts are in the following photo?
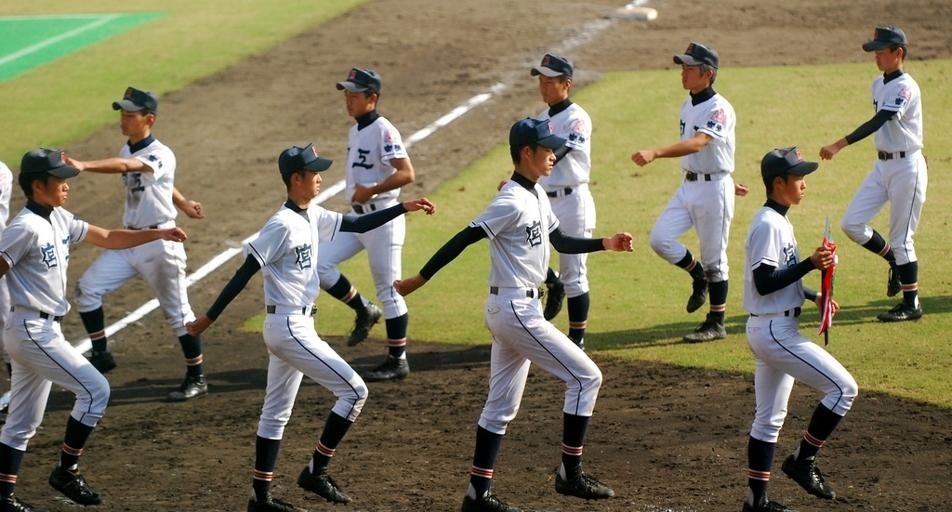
[685,174,710,182]
[546,188,573,199]
[351,203,376,214]
[127,226,157,229]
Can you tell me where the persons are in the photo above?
[187,143,433,510]
[317,63,413,380]
[1,145,185,511]
[393,118,633,511]
[61,86,207,399]
[0,163,11,415]
[497,54,597,352]
[632,41,746,340]
[743,143,858,512]
[819,23,931,323]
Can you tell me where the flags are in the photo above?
[819,239,836,338]
